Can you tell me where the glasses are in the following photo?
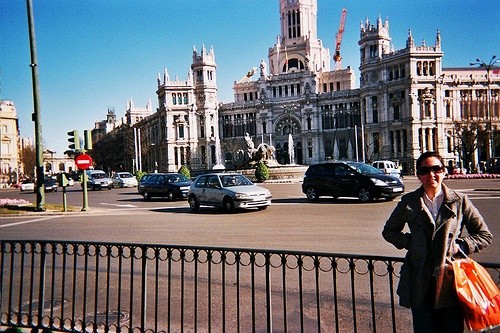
[418,166,444,175]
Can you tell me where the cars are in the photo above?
[137,173,191,201]
[112,172,138,188]
[34,177,58,194]
[19,179,35,192]
[68,177,75,187]
[187,172,273,211]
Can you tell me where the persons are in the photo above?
[382,152,493,333]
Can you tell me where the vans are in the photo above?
[81,170,114,191]
[301,161,406,202]
[371,160,401,180]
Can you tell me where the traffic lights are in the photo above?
[66,129,81,150]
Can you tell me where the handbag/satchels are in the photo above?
[446,244,500,333]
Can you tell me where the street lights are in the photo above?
[469,54,500,158]
[150,142,159,173]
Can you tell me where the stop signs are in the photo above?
[75,153,92,170]
